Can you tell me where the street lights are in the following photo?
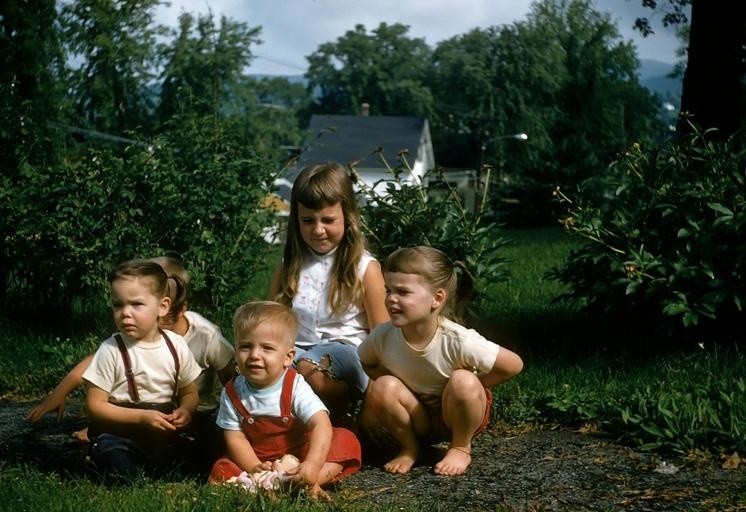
[478,131,528,180]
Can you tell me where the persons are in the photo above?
[25,256,237,442]
[266,159,389,432]
[59,259,221,491]
[356,245,525,476]
[207,304,362,502]
